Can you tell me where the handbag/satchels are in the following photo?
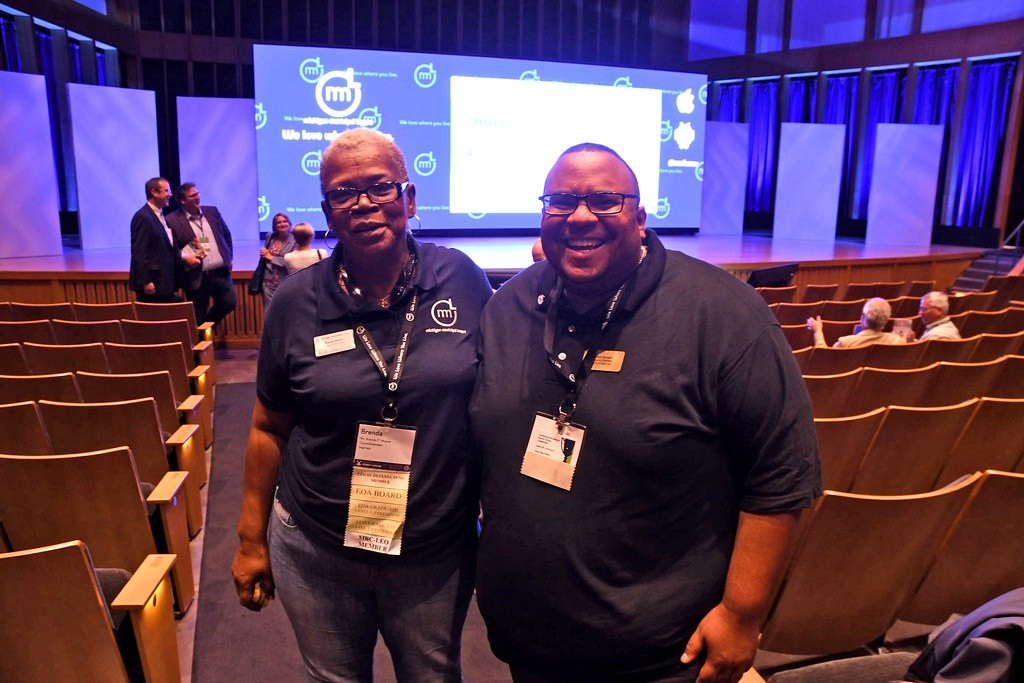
[247,235,274,295]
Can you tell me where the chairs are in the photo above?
[0,540,183,683]
[0,302,13,321]
[0,400,54,456]
[104,342,215,451]
[76,370,208,489]
[72,301,137,321]
[11,301,76,321]
[121,318,215,413]
[38,396,203,538]
[760,273,1024,683]
[134,301,217,386]
[0,342,33,376]
[0,319,56,345]
[0,446,196,615]
[23,341,112,376]
[0,371,84,405]
[51,319,125,345]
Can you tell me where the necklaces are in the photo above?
[339,253,416,306]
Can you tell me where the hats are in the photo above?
[862,297,892,325]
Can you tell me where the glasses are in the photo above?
[324,179,410,210]
[184,191,200,198]
[537,192,641,217]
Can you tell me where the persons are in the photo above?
[283,223,326,275]
[805,297,907,350]
[128,177,184,303]
[902,291,961,344]
[465,143,824,683]
[532,239,546,262]
[163,182,238,329]
[231,127,494,683]
[258,213,299,316]
